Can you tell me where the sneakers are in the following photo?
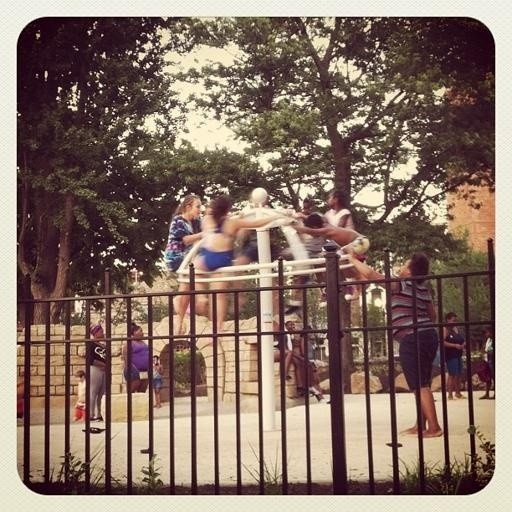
[90,416,103,421]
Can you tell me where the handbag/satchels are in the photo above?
[138,371,149,379]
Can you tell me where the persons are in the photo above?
[274,321,323,401]
[165,188,369,343]
[122,322,149,393]
[349,253,442,437]
[479,325,494,399]
[75,370,86,422]
[153,355,164,408]
[442,313,467,400]
[90,324,107,421]
